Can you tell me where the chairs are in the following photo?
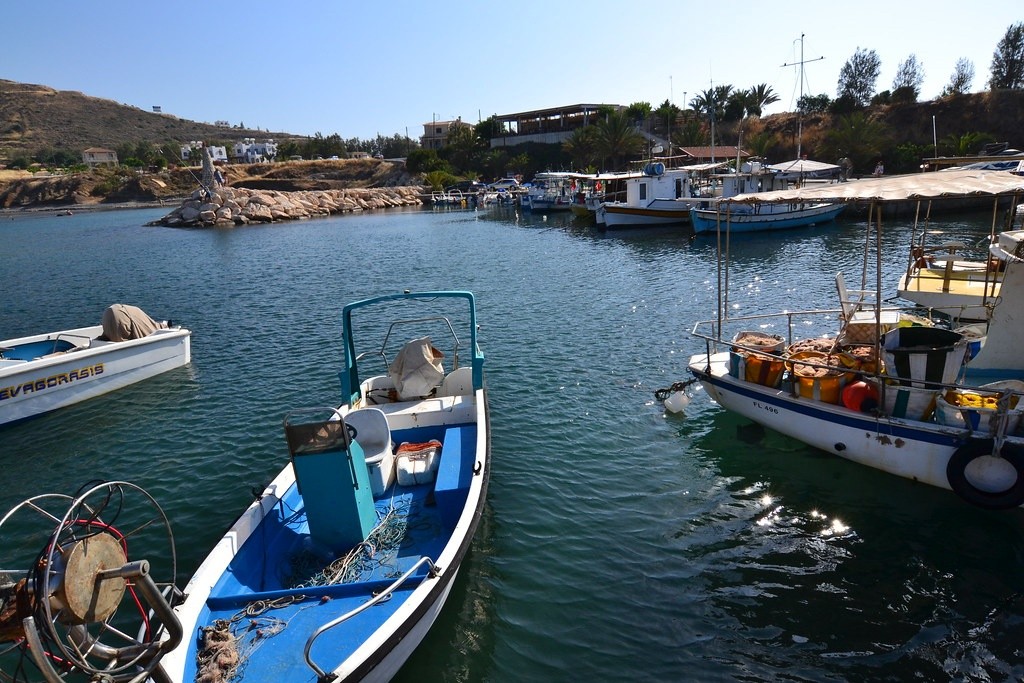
[341,408,391,464]
[835,272,900,325]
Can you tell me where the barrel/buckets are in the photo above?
[644,162,665,175]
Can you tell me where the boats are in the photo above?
[0,289,492,683]
[0,322,192,423]
[424,71,1024,514]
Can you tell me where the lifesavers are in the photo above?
[947,438,1023,514]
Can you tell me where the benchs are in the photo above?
[435,427,476,524]
[363,394,476,432]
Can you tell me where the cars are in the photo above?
[360,154,369,159]
[374,154,384,159]
